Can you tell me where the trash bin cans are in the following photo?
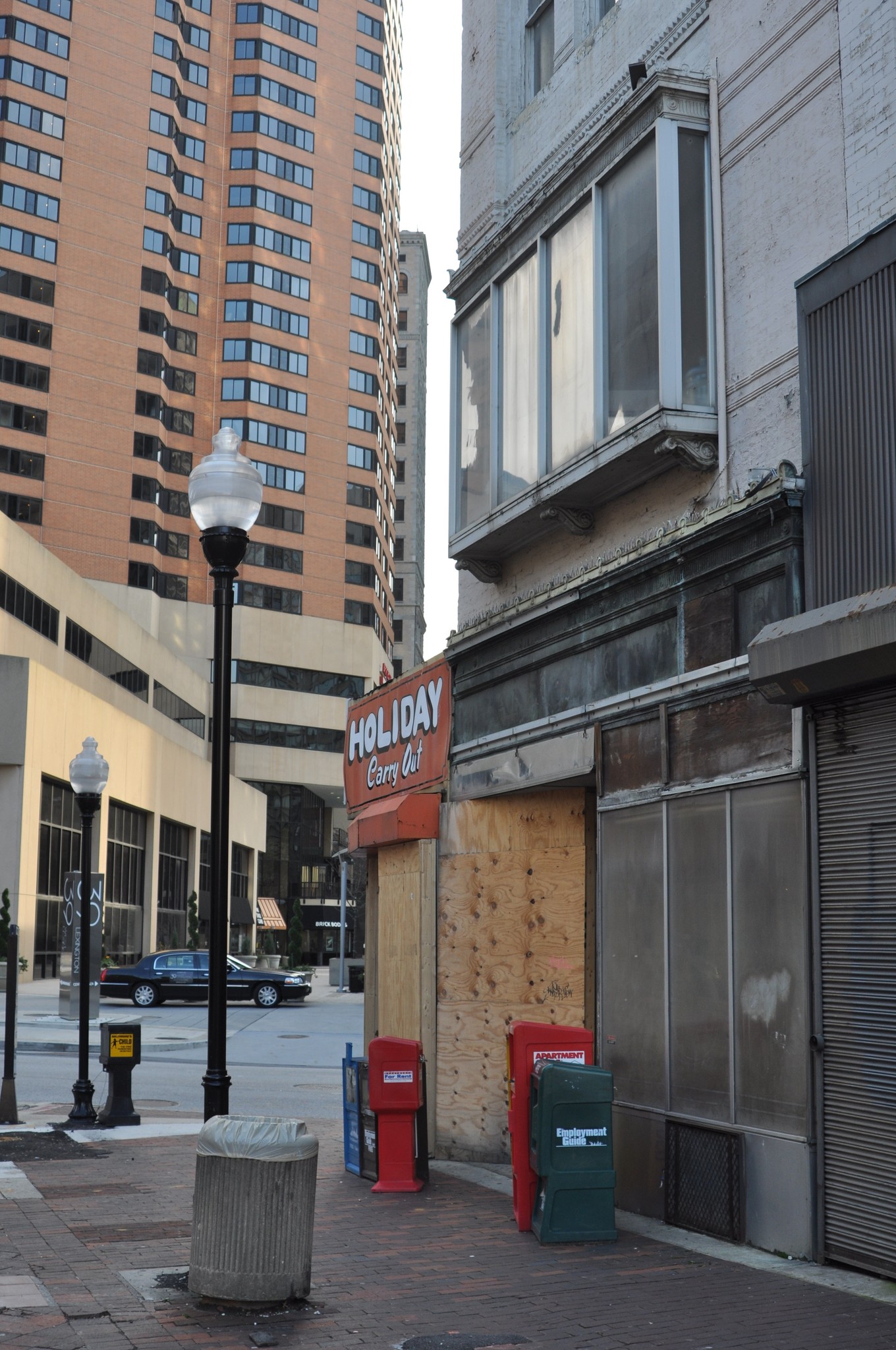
[347,965,364,992]
[188,1115,318,1308]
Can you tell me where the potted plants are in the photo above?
[100,956,120,975]
[234,938,290,969]
[0,888,29,992]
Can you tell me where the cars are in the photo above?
[97,950,313,1009]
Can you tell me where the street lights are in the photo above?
[68,737,109,1124]
[186,421,266,1129]
